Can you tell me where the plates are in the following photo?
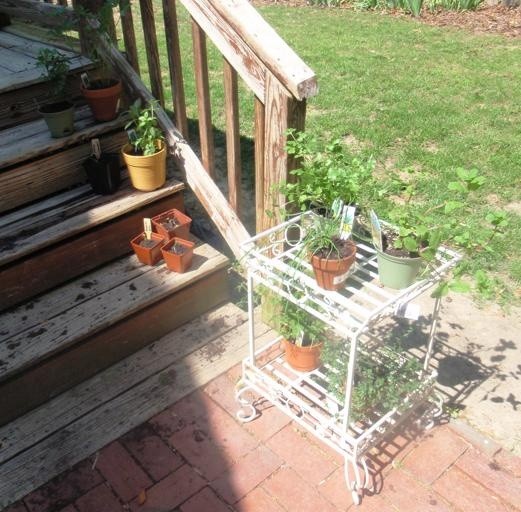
[229,209,460,507]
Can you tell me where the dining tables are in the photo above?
[288,215,358,293]
[33,47,79,139]
[258,281,327,374]
[320,332,429,424]
[271,122,378,241]
[124,97,168,193]
[50,1,132,121]
[371,204,457,289]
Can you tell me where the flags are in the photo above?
[130,231,165,267]
[81,152,121,196]
[152,209,192,242]
[161,236,196,272]
